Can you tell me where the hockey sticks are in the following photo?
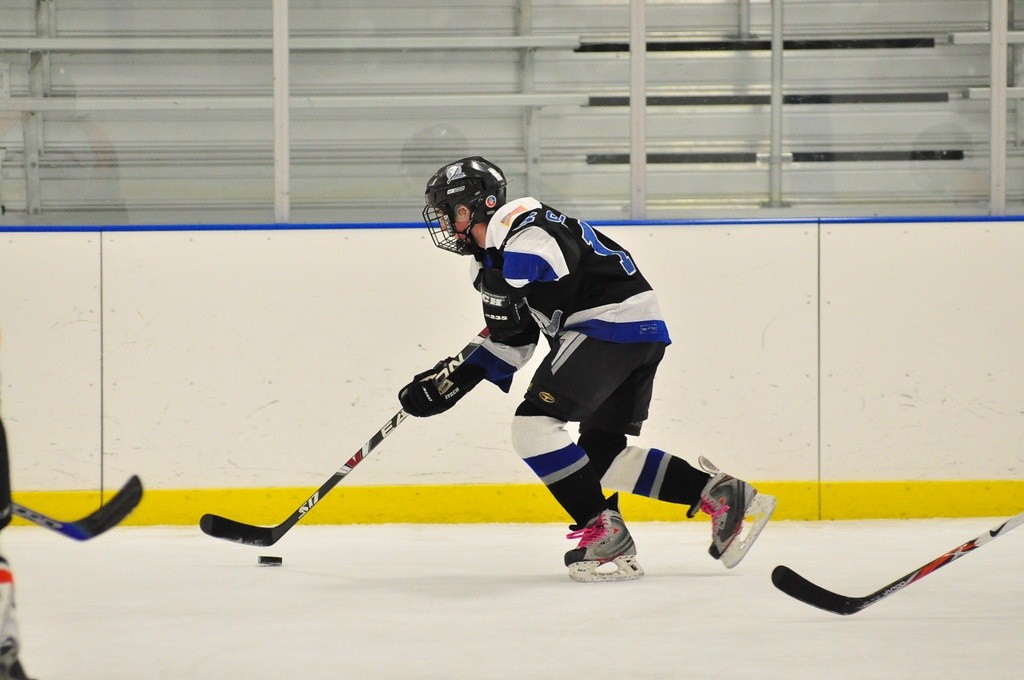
[198,299,528,548]
[769,512,1024,616]
[9,473,144,542]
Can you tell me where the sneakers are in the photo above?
[564,493,645,583]
[687,457,776,569]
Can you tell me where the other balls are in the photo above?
[258,555,283,567]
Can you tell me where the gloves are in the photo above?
[478,269,539,347]
[397,356,484,417]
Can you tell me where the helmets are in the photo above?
[425,156,507,255]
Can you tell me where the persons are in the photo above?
[397,153,779,585]
[0,416,38,680]
